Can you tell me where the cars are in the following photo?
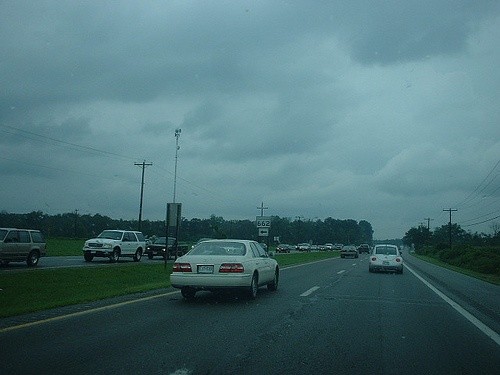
[276,244,291,253]
[170,239,280,301]
[340,246,359,259]
[369,244,403,274]
[259,243,267,251]
[196,238,245,255]
[289,243,344,252]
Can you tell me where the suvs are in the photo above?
[0,228,47,268]
[82,229,147,264]
[358,244,370,255]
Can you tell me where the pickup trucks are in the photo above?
[147,236,188,261]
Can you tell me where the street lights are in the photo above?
[173,128,182,203]
